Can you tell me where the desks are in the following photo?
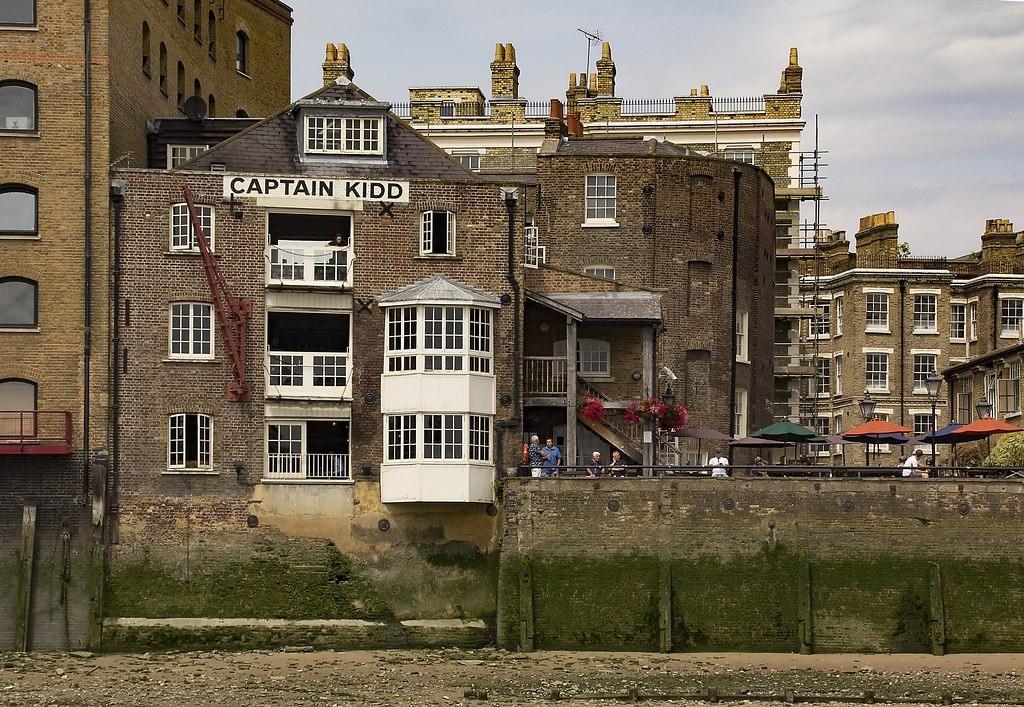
[672,468,1013,478]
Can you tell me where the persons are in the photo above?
[539,437,562,477]
[333,234,347,280]
[708,448,730,477]
[607,450,626,477]
[586,452,606,478]
[749,455,770,477]
[902,449,931,479]
[528,435,550,477]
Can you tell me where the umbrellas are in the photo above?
[806,418,913,466]
[949,417,1024,467]
[750,418,816,456]
[917,420,991,467]
[662,427,735,466]
[728,428,796,458]
[898,431,932,446]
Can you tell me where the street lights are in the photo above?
[976,393,993,421]
[859,389,877,465]
[924,366,942,468]
